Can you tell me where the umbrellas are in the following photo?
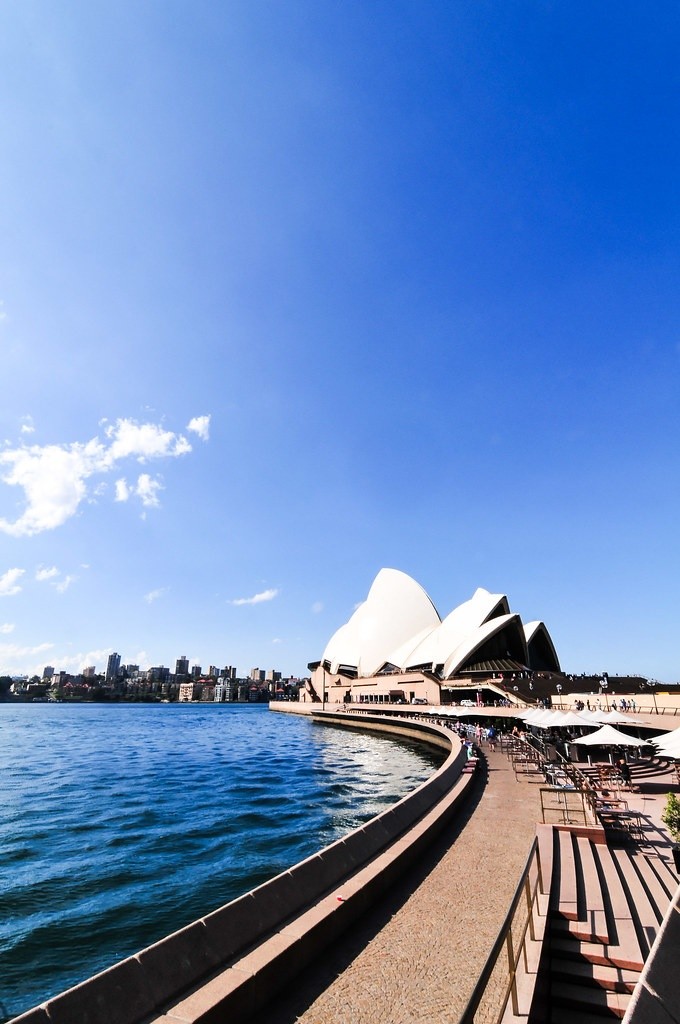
[569,724,652,745]
[517,707,637,727]
[647,728,680,759]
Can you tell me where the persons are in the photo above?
[595,699,601,710]
[611,699,635,710]
[475,724,482,747]
[575,699,590,710]
[487,724,496,752]
[444,720,460,733]
[619,759,630,780]
[537,698,551,709]
[494,699,502,706]
[512,726,526,739]
